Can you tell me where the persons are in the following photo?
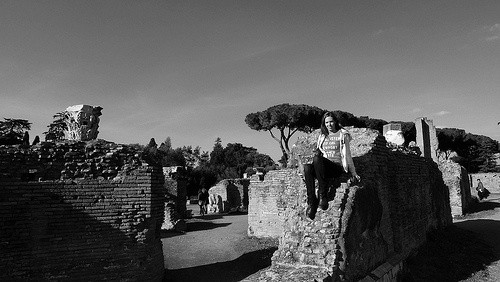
[201,187,209,214]
[476,178,487,199]
[303,113,361,220]
[196,189,205,216]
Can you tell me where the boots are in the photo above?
[317,189,330,207]
[306,196,318,218]
